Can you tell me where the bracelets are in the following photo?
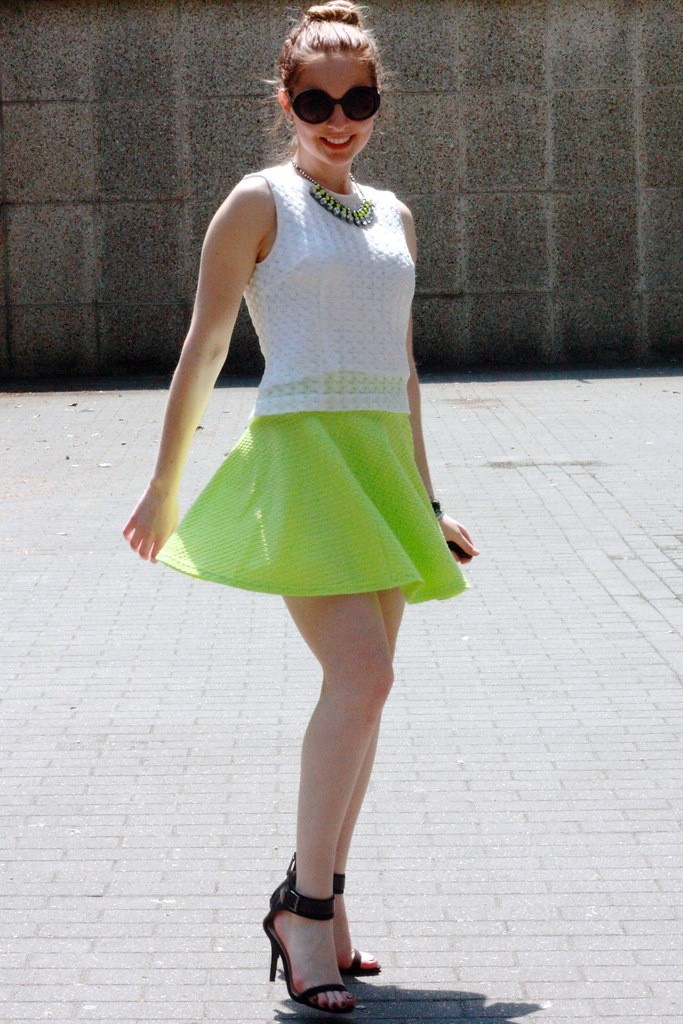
[428,497,442,519]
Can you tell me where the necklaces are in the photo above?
[291,154,375,226]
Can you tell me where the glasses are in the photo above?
[286,83,382,125]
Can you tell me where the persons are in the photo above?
[122,0,481,1012]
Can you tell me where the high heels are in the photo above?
[262,851,382,1015]
